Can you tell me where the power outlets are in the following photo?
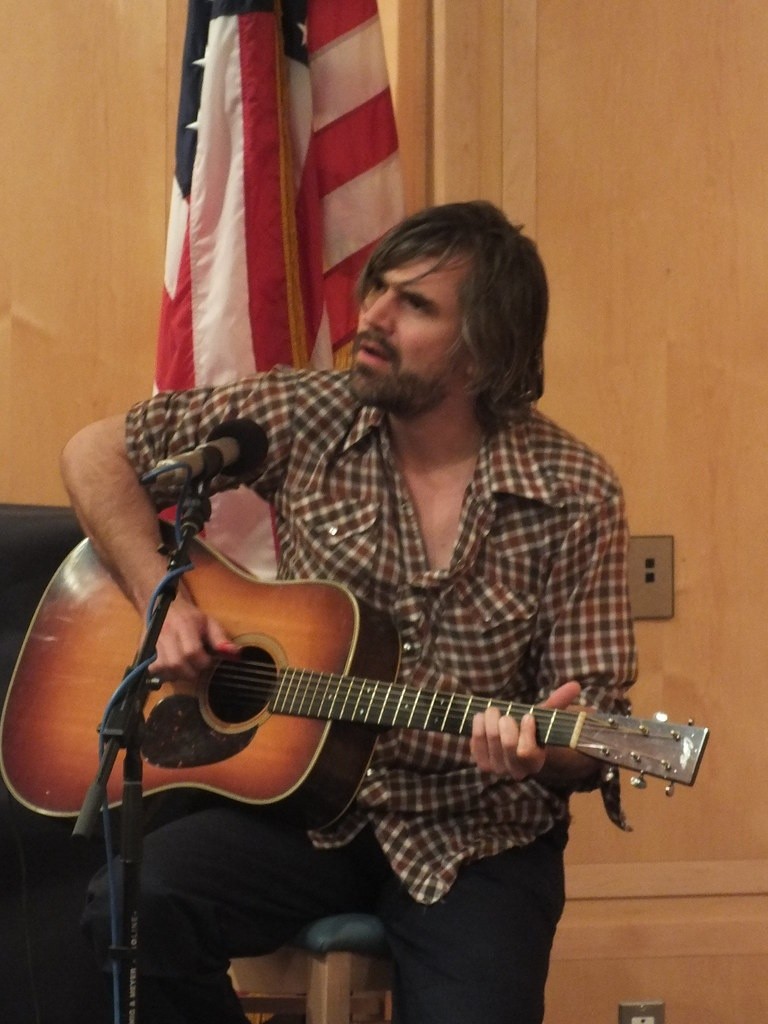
[620,1001,667,1024]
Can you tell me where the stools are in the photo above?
[226,911,406,1024]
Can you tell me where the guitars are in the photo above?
[0,518,711,831]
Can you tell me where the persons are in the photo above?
[62,201,640,1024]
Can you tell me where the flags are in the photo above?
[161,1,406,582]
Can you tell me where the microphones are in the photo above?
[139,419,269,485]
